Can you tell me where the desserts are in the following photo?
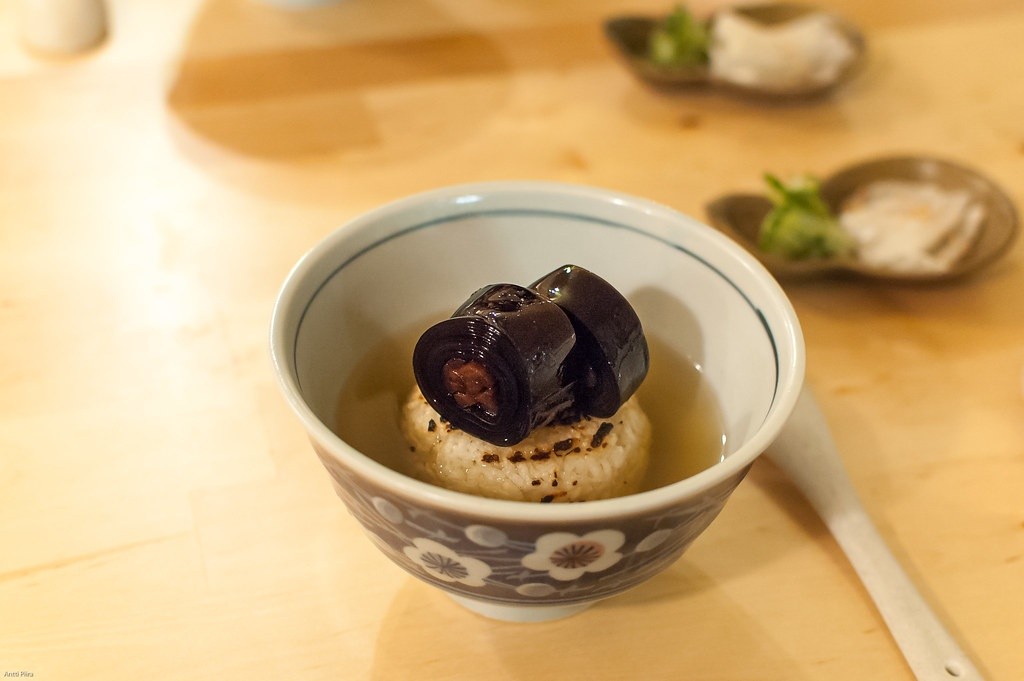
[338,261,722,500]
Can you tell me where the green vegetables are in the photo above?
[662,6,695,51]
[756,168,835,222]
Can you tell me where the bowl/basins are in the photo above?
[269,182,808,626]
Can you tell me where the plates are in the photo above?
[605,1,876,98]
[707,150,1019,278]
